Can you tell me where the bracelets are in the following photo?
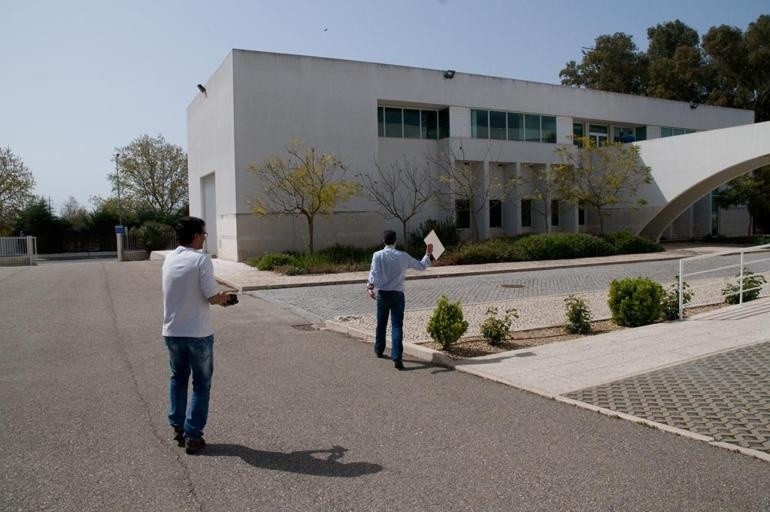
[367,282,374,289]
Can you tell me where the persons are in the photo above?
[161,215,239,454]
[366,229,434,369]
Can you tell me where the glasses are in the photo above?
[201,232,208,238]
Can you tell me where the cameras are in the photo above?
[227,293,239,305]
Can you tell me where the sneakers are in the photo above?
[377,353,383,358]
[172,425,205,455]
[395,360,404,368]
